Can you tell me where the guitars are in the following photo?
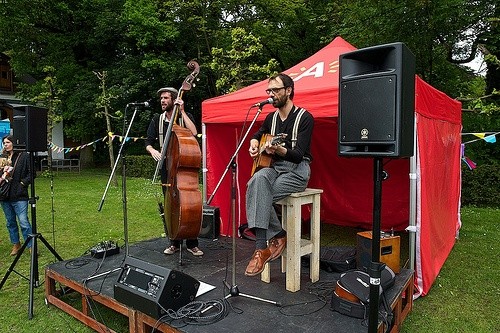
[250,132,289,177]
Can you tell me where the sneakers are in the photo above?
[163,245,181,255]
[186,247,204,256]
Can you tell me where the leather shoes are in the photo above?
[29,248,40,256]
[244,247,272,276]
[267,230,287,260]
[10,245,22,255]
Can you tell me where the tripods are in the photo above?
[194,108,283,316]
[0,153,63,320]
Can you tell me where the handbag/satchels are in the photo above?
[0,176,12,202]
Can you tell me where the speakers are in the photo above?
[338,42,414,157]
[113,256,202,315]
[356,231,400,274]
[198,206,220,239]
[12,106,48,153]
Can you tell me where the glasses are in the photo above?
[266,86,286,94]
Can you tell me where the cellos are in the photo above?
[150,59,205,265]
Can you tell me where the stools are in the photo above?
[261,188,324,292]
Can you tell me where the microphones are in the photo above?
[128,101,150,107]
[255,98,275,107]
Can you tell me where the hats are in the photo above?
[157,87,181,95]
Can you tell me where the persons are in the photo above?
[146,88,204,256]
[0,136,37,256]
[244,74,314,276]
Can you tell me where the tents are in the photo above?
[201,36,462,300]
